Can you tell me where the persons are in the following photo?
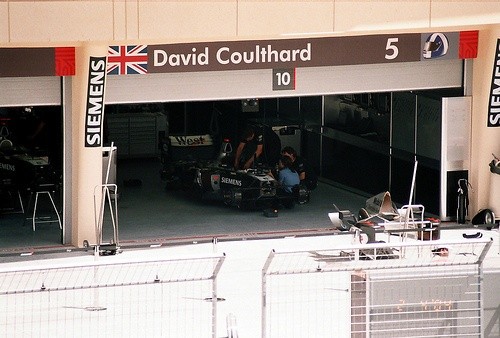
[233,126,316,208]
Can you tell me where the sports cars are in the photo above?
[172,151,311,211]
[0,122,64,215]
